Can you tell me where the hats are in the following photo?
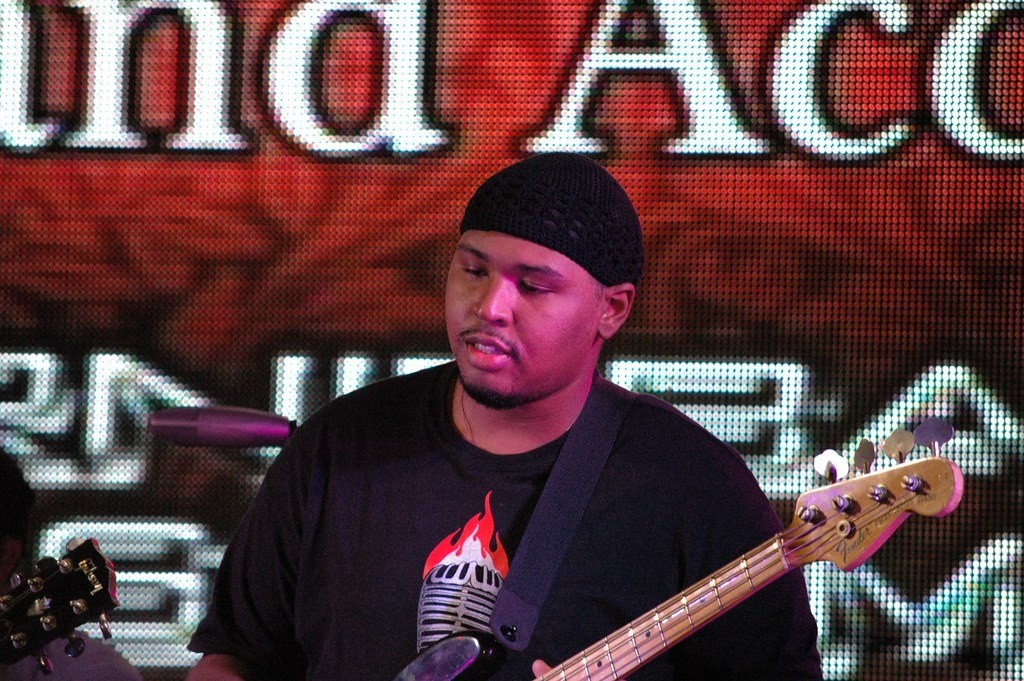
[462,151,644,293]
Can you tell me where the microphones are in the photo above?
[148,406,297,448]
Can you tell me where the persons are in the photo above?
[0,447,144,681]
[187,152,823,681]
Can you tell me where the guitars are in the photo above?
[392,416,967,681]
[0,534,120,680]
